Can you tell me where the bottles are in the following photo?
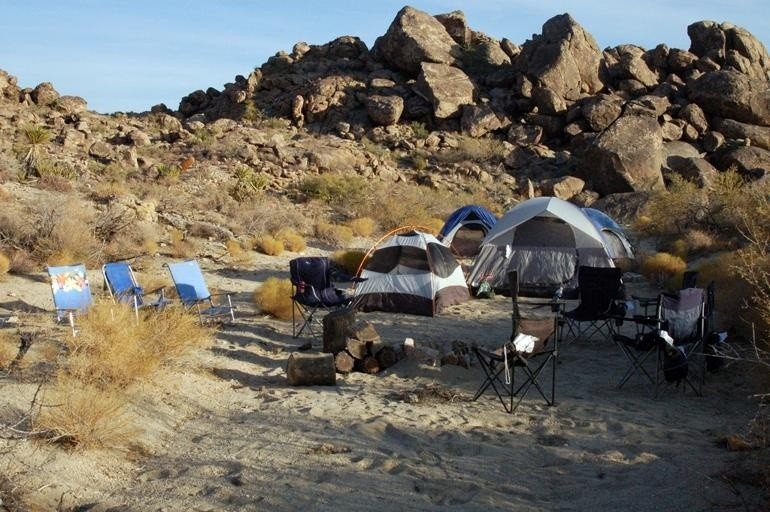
[552,286,564,302]
[297,281,305,293]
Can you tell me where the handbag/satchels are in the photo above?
[513,332,540,353]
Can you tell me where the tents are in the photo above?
[345,196,638,318]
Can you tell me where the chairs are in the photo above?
[162,258,239,326]
[289,256,354,344]
[470,265,715,414]
[42,262,95,337]
[101,262,174,326]
